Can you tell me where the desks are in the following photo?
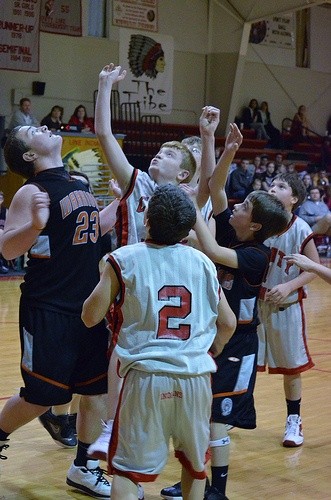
[4,132,127,208]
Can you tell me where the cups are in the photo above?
[240,122,244,130]
[324,130,328,136]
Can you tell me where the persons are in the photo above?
[258,101,280,136]
[229,158,252,198]
[242,99,270,140]
[290,105,309,141]
[41,106,64,130]
[1,98,40,149]
[82,185,237,500]
[69,105,95,134]
[242,154,331,211]
[256,172,320,449]
[0,126,112,499]
[299,188,331,224]
[282,253,331,285]
[40,136,213,447]
[88,62,220,461]
[160,123,287,500]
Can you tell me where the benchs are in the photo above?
[91,118,323,206]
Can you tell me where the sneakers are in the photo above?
[66,459,144,500]
[160,477,210,500]
[203,486,229,500]
[86,420,113,462]
[282,414,304,447]
[38,407,77,448]
[225,424,233,431]
